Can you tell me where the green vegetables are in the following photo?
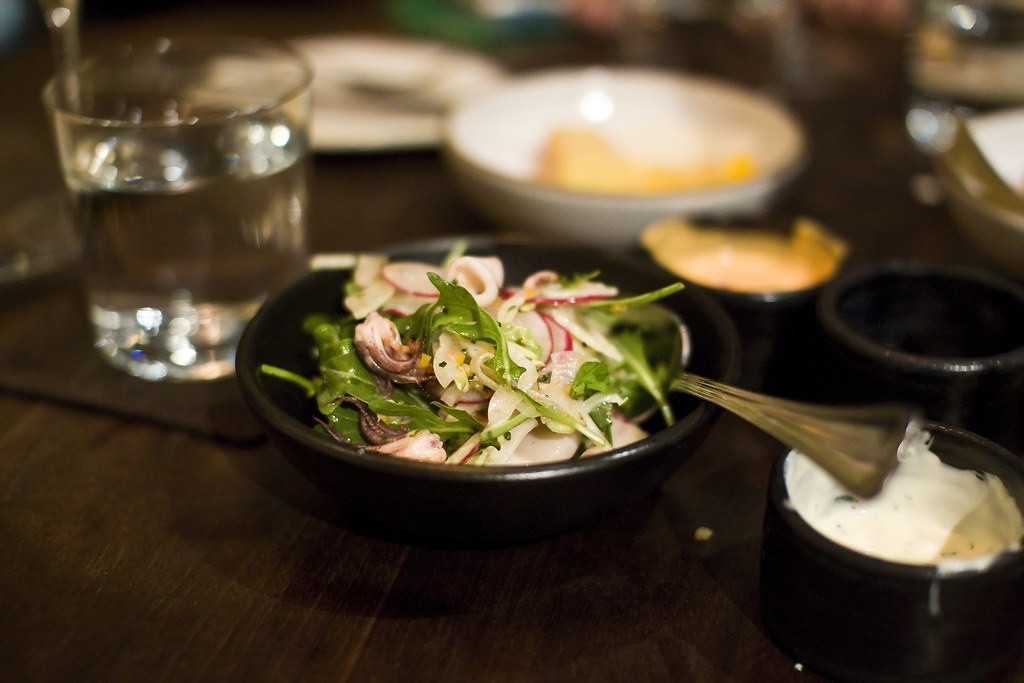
[261,236,695,458]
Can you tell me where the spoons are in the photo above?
[627,302,910,500]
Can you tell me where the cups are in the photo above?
[39,28,309,381]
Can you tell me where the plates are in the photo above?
[210,32,499,153]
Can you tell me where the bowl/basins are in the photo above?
[234,232,740,551]
[757,418,1024,683]
[445,67,804,263]
[814,262,1024,458]
